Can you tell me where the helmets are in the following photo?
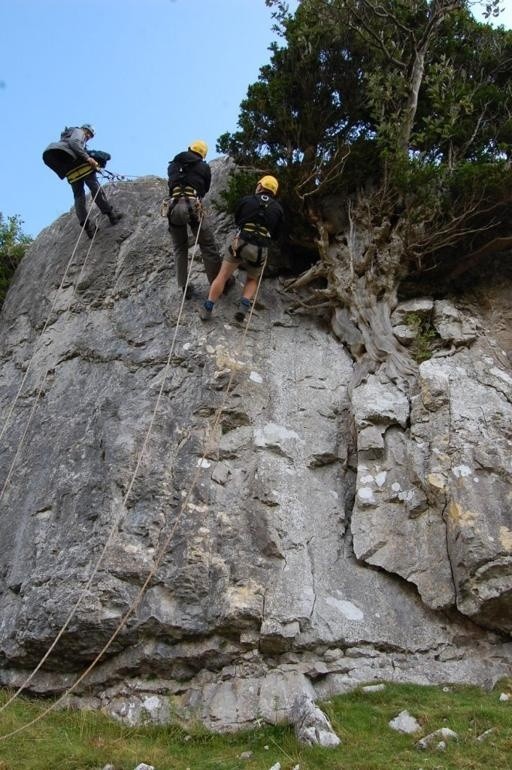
[255,175,279,195]
[189,139,209,160]
[80,124,96,140]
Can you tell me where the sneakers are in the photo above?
[181,281,195,300]
[86,223,100,239]
[225,274,235,288]
[198,302,213,320]
[236,297,252,320]
[107,210,124,225]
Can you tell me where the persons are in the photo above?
[195,174,284,322]
[165,138,236,301]
[58,123,125,239]
